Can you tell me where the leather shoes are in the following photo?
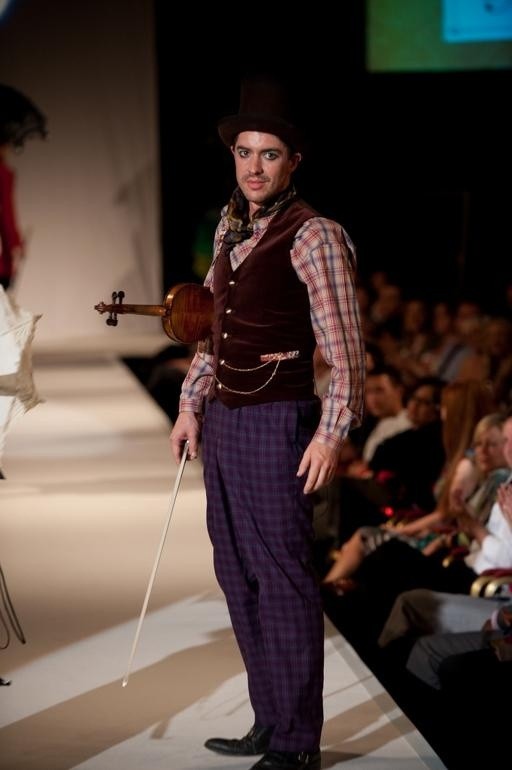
[205,722,274,755]
[249,748,322,769]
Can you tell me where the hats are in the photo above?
[217,79,300,153]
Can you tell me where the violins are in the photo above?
[94,285,215,342]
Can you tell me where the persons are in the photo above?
[158,266,511,694]
[171,128,368,769]
